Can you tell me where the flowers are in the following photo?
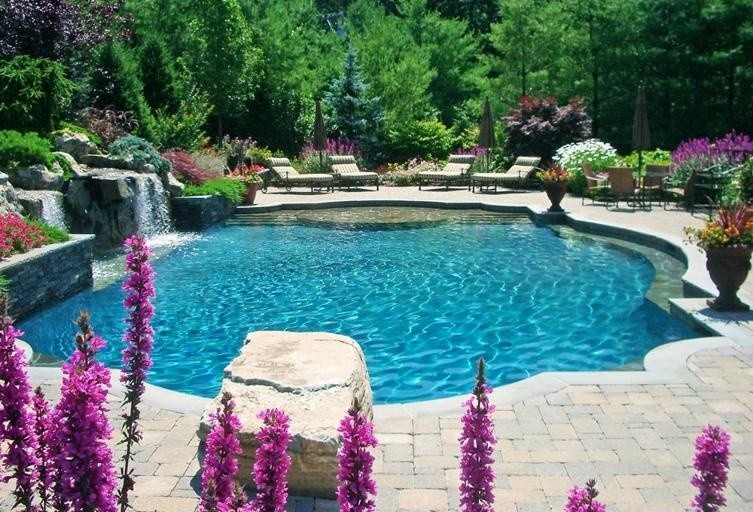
[536,163,572,182]
[226,163,270,185]
[681,194,753,253]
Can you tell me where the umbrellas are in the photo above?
[478,94,497,174]
[631,80,652,188]
[313,99,328,172]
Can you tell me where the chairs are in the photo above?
[260,157,334,193]
[329,155,380,192]
[581,162,725,218]
[473,156,542,193]
[418,154,475,191]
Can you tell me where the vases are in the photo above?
[705,243,753,312]
[543,180,569,212]
[243,181,259,205]
